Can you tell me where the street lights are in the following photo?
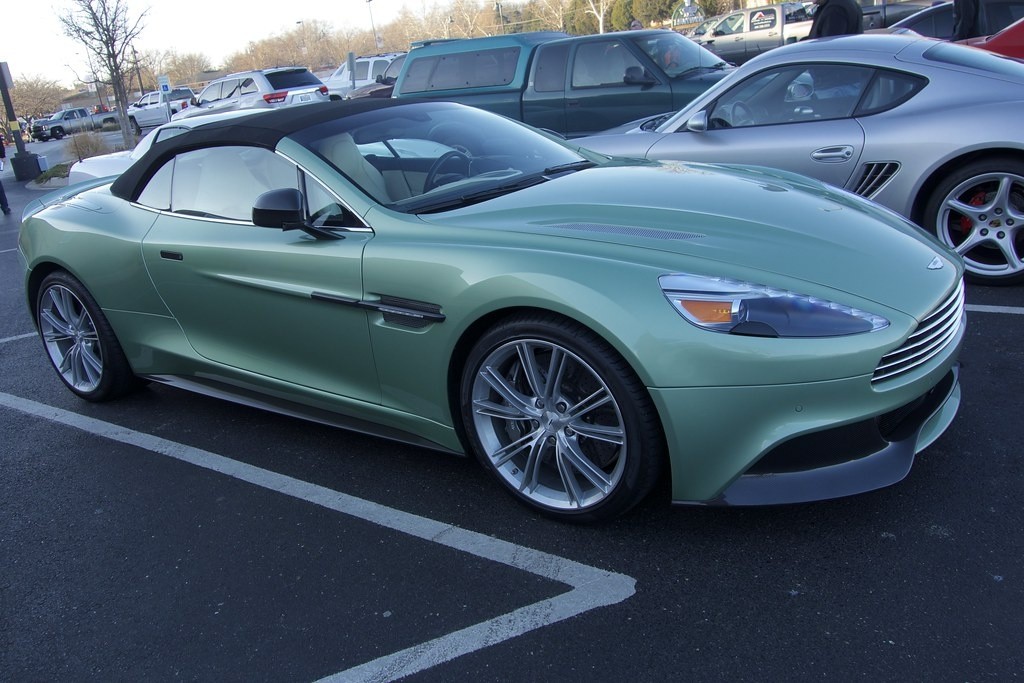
[296,20,308,67]
[366,0,379,53]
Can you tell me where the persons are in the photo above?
[949,0,1015,42]
[0,136,16,215]
[804,0,864,90]
[631,20,644,29]
[651,40,680,70]
[26,123,35,143]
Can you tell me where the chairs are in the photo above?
[856,72,910,111]
[194,147,265,220]
[572,44,640,87]
[304,131,391,217]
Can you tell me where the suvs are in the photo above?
[170,66,330,122]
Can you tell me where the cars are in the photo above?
[67,106,281,191]
[343,54,410,99]
[949,16,1024,59]
[888,0,1024,42]
[323,51,404,101]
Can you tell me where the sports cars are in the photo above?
[16,93,971,530]
[569,32,1024,286]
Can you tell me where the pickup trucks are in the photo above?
[127,87,198,136]
[388,23,817,140]
[680,3,815,67]
[30,107,120,140]
